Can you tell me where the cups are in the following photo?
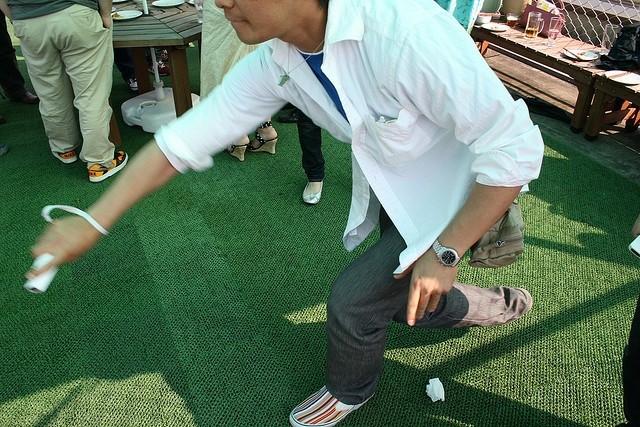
[547,17,563,48]
[601,24,619,56]
[194,1,203,23]
[524,11,544,39]
[507,13,520,27]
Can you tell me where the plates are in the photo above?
[111,6,116,13]
[482,22,508,32]
[111,10,142,20]
[152,0,185,8]
[563,50,599,62]
[604,70,640,84]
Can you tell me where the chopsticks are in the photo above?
[608,71,633,79]
[563,47,583,61]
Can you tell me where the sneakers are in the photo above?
[288,385,374,426]
[127,77,138,92]
[19,91,39,104]
[147,61,169,76]
[303,181,324,205]
[87,150,128,183]
[52,150,77,163]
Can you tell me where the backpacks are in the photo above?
[596,22,639,70]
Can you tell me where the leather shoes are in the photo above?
[278,109,299,123]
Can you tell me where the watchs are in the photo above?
[432,239,460,268]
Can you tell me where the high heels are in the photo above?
[229,145,247,162]
[248,122,278,155]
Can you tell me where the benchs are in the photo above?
[471,12,610,134]
[584,71,640,142]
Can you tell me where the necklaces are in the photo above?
[277,37,326,87]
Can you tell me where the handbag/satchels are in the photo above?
[469,199,525,268]
[518,0,566,40]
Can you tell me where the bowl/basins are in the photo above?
[474,16,492,24]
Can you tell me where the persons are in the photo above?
[631,214,640,239]
[114,47,139,91]
[295,107,326,206]
[200,0,280,162]
[24,0,545,426]
[147,46,170,76]
[0,10,38,125]
[0,0,129,183]
